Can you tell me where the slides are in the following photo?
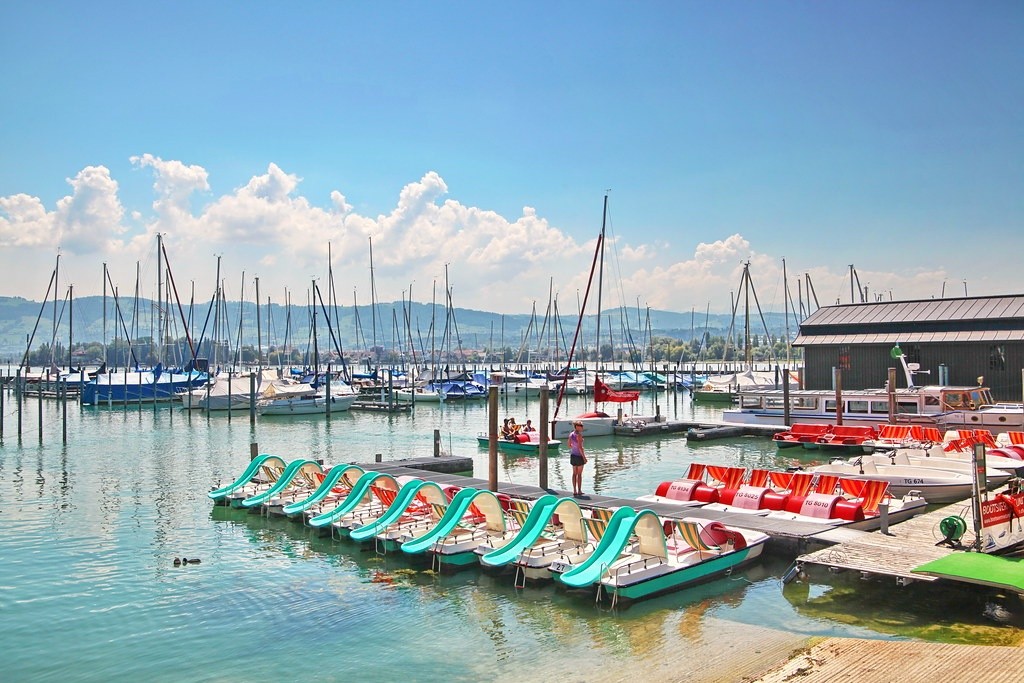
[282,463,365,515]
[207,454,286,498]
[348,480,447,541]
[557,505,667,587]
[242,460,323,507]
[482,494,587,566]
[309,471,400,527]
[399,487,506,555]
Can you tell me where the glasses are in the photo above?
[577,425,584,427]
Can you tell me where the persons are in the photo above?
[501,417,517,440]
[523,420,532,433]
[568,421,587,496]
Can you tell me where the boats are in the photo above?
[559,505,773,603]
[0,193,1024,594]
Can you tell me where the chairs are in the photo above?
[671,519,722,563]
[261,465,638,553]
[878,424,1024,454]
[684,463,896,516]
